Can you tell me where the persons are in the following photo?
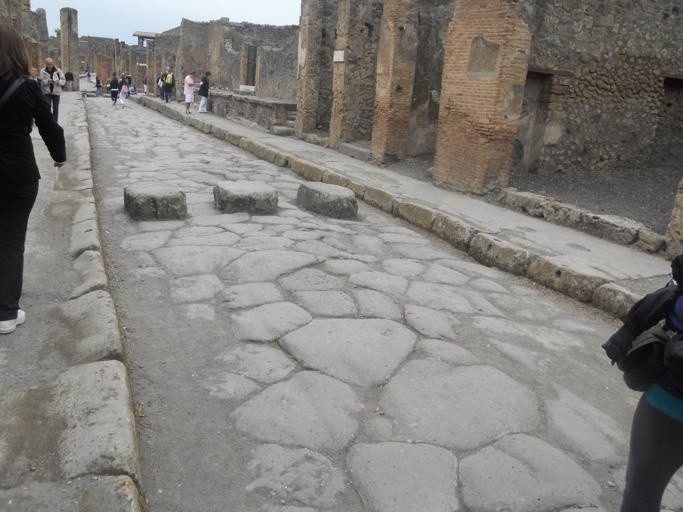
[106,68,212,113]
[615,174,683,512]
[30,67,39,83]
[38,58,66,122]
[0,19,67,334]
[65,71,73,92]
[96,74,102,97]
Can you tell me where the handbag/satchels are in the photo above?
[616,318,668,392]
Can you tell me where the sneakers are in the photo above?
[0,308,27,334]
